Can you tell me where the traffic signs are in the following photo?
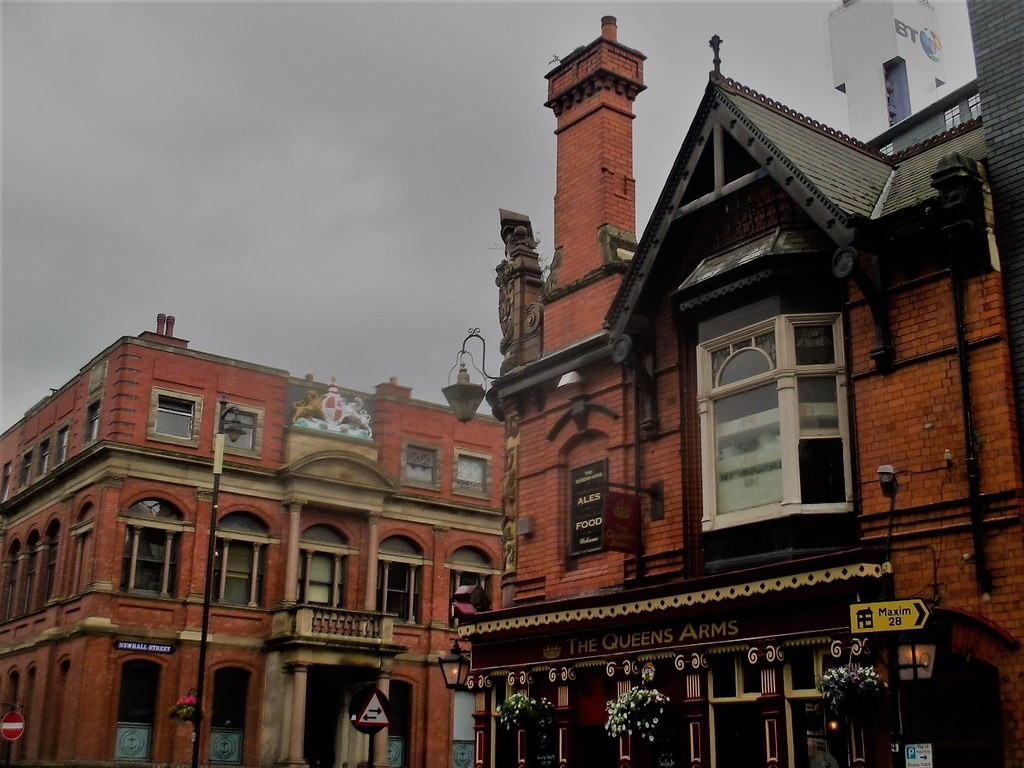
[850,597,931,639]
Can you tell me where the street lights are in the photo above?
[192,395,249,767]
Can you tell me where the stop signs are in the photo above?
[1,711,25,741]
